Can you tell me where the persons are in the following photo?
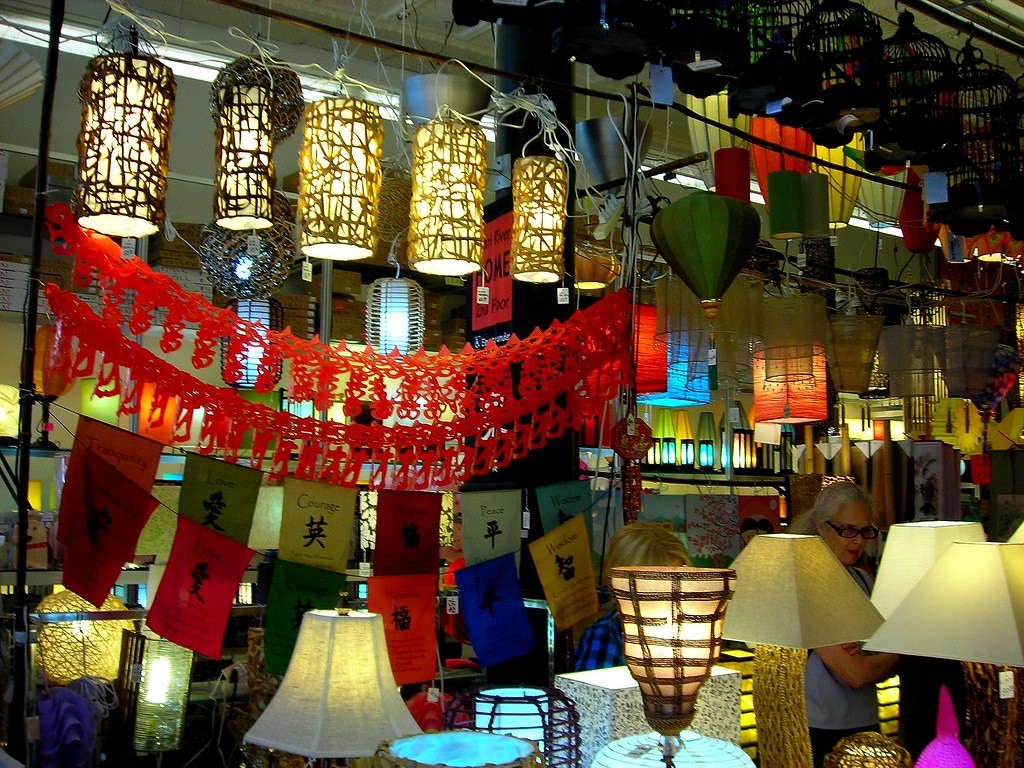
[803,479,899,768]
[569,522,690,673]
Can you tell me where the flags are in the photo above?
[55,415,600,680]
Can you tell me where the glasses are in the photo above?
[826,520,879,540]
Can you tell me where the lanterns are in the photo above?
[587,85,1024,485]
[77,54,621,392]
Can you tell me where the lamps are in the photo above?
[575,116,653,197]
[766,170,806,241]
[826,314,886,394]
[69,32,178,239]
[363,256,425,360]
[212,82,275,232]
[715,146,750,204]
[509,155,568,283]
[402,72,494,126]
[646,427,800,477]
[802,172,831,240]
[406,110,487,278]
[934,315,1005,398]
[752,342,828,425]
[31,519,1024,768]
[197,193,305,390]
[574,66,621,290]
[297,91,384,261]
[634,302,668,396]
[877,313,948,398]
